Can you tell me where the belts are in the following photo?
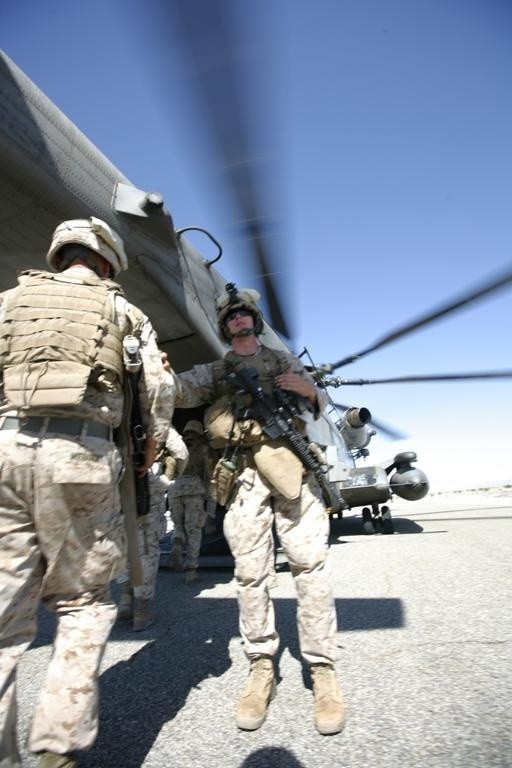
[0,414,117,442]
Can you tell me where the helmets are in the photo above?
[182,418,204,435]
[42,215,130,278]
[212,287,264,343]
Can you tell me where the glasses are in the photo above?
[221,309,255,326]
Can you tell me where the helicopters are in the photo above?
[1,3,512,535]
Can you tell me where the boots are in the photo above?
[169,537,185,571]
[234,653,277,730]
[309,660,347,735]
[184,566,200,585]
[116,585,166,632]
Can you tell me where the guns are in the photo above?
[122,334,150,554]
[235,367,349,510]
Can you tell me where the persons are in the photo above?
[128,423,189,632]
[169,283,344,737]
[0,214,177,768]
[170,419,216,586]
